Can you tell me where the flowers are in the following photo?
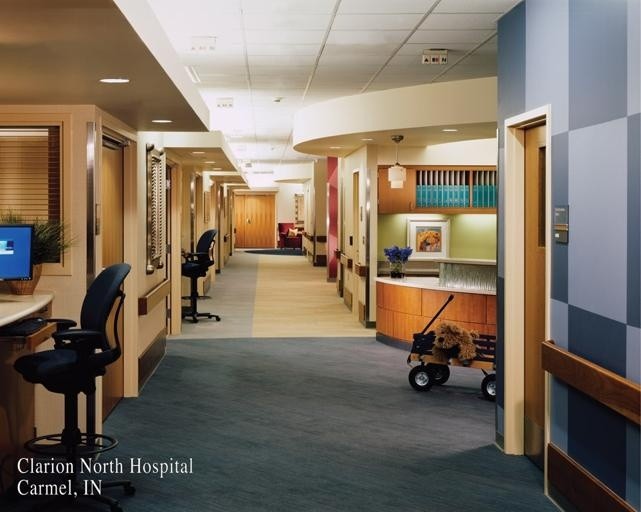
[383,242,415,272]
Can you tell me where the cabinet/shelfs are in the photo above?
[376,164,497,215]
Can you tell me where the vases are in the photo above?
[389,258,407,280]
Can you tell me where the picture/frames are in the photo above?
[406,217,451,262]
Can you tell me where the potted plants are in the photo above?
[0,202,82,295]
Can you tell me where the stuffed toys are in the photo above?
[433,323,479,363]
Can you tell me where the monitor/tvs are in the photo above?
[0,225,36,280]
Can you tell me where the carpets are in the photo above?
[1,336,565,512]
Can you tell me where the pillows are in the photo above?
[287,227,299,237]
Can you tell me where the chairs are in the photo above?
[13,261,134,510]
[277,221,303,251]
[182,228,224,324]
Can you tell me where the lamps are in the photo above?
[387,135,409,189]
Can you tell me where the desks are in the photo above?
[1,292,53,491]
